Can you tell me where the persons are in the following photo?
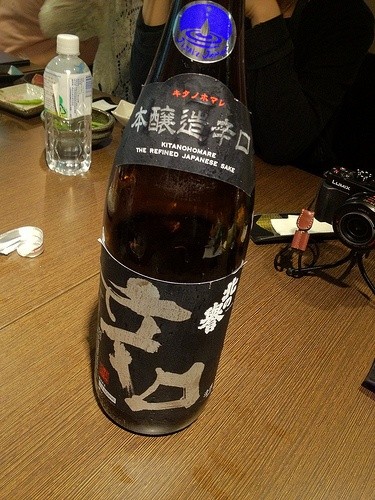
[129,0,375,177]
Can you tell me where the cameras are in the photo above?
[315,167,375,252]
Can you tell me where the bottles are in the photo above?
[93,1,257,436]
[43,32,93,176]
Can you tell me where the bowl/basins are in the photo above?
[40,105,115,147]
[110,99,136,127]
[0,83,46,117]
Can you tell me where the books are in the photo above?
[0,50,45,86]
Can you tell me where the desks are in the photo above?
[0,85,375,500]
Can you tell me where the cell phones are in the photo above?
[251,212,338,244]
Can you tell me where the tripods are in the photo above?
[287,249,375,295]
